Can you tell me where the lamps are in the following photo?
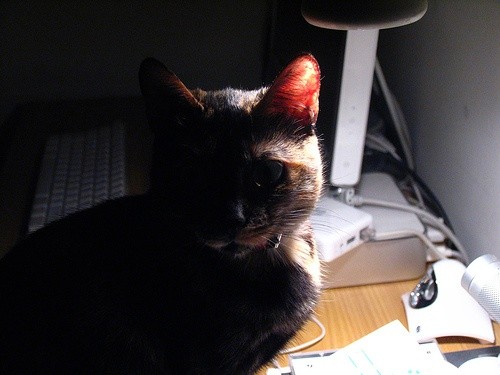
[297,0,427,288]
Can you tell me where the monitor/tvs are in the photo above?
[251,1,381,185]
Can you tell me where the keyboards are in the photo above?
[28,122,128,232]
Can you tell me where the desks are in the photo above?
[1,98,500,375]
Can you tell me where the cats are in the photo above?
[1,49,326,375]
